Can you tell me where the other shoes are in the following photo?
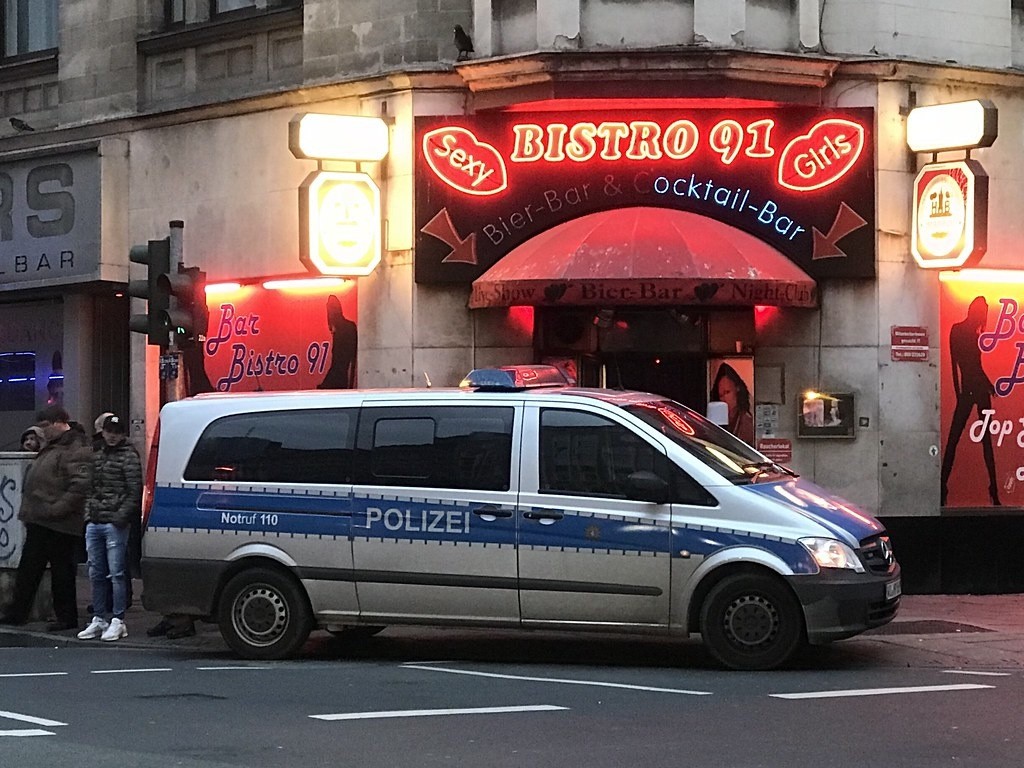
[47,620,78,631]
[147,620,174,636]
[0,616,28,626]
[167,621,196,638]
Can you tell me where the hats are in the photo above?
[104,415,125,434]
[94,412,115,434]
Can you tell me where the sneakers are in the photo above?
[77,616,109,639]
[101,618,128,641]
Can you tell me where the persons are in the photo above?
[317,293,357,390]
[0,405,144,642]
[710,361,754,449]
[146,613,195,640]
[940,295,1001,507]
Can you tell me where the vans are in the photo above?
[138,361,903,676]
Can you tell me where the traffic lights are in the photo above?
[120,238,207,355]
